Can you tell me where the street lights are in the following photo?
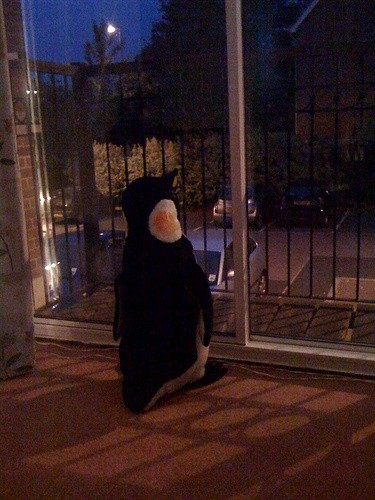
[107,24,124,63]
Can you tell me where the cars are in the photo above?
[283,180,330,226]
[214,182,267,226]
[48,230,125,313]
[180,229,270,297]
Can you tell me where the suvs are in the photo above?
[50,187,115,222]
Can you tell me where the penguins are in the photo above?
[113,167,216,414]
